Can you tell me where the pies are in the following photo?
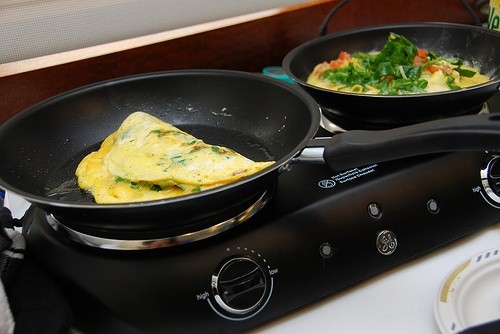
[75,111,278,205]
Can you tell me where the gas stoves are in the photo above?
[0,93,500,333]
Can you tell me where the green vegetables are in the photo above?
[320,32,477,94]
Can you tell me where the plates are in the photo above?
[433,247,500,333]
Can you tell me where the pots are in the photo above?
[283,22,500,130]
[0,69,500,225]
